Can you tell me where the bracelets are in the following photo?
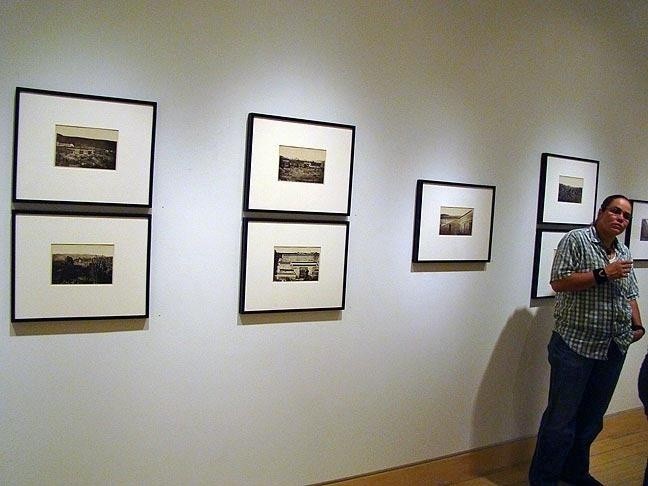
[630,325,646,336]
[592,268,608,284]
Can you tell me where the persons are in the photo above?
[527,192,644,485]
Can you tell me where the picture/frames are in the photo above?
[537,153,600,227]
[11,210,152,322]
[412,179,496,264]
[624,199,648,261]
[239,218,350,314]
[532,229,573,299]
[13,84,157,207]
[243,113,355,216]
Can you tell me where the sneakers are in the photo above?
[566,471,603,485]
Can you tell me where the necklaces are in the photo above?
[597,234,612,260]
